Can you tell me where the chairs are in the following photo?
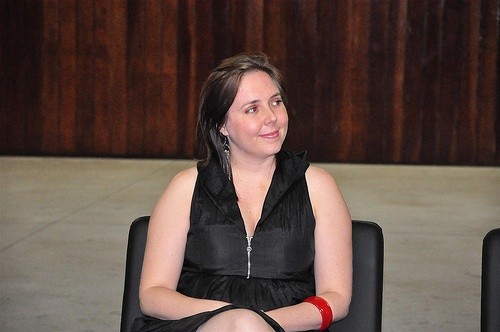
[121,215,385,331]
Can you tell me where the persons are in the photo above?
[129,54,352,332]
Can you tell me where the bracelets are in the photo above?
[304,297,332,331]
